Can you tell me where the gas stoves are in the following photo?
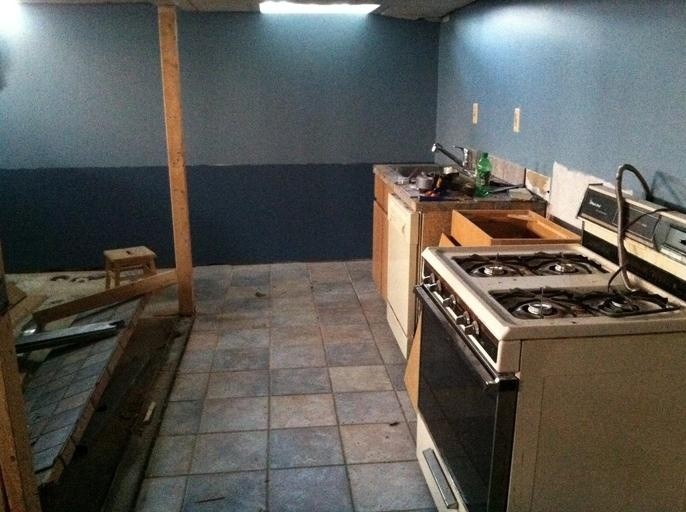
[451,252,679,321]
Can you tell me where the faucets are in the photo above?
[430,142,472,170]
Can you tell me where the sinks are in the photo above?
[388,163,441,178]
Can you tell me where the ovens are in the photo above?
[412,258,521,511]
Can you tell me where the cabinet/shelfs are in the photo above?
[371,175,395,302]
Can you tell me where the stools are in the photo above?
[104,245,156,290]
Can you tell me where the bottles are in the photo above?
[474,152,493,198]
